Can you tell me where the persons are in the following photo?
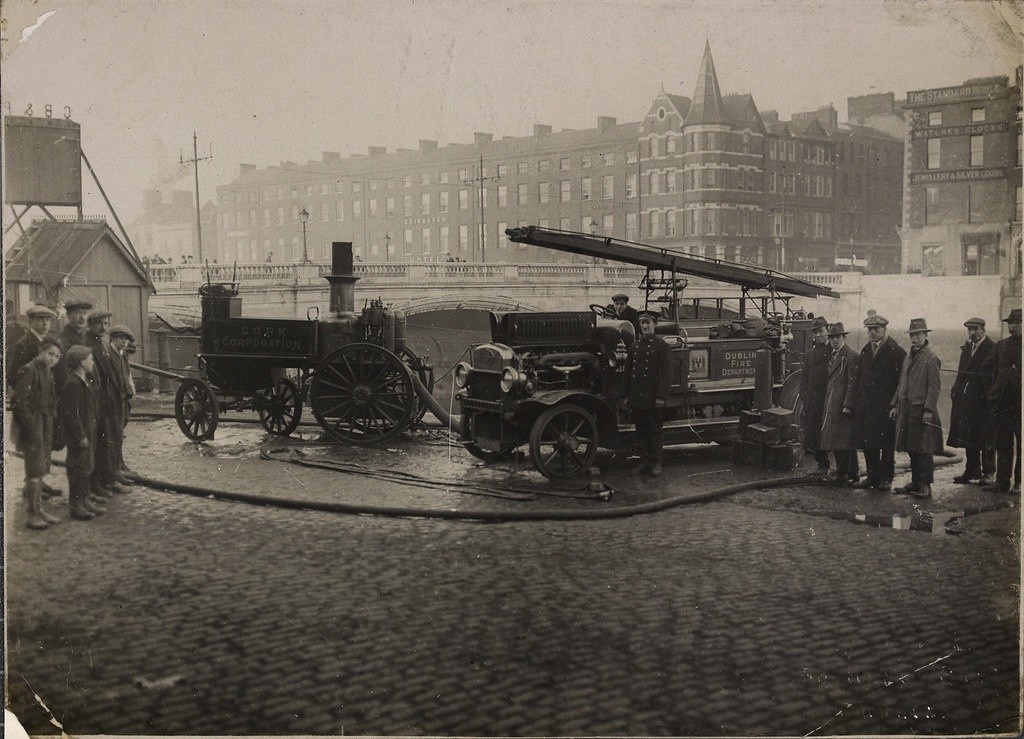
[799,316,833,475]
[6,305,63,499]
[52,300,94,450]
[10,335,62,530]
[854,314,906,491]
[819,322,862,483]
[187,255,193,264]
[356,256,368,272]
[946,317,996,486]
[86,309,133,496]
[979,308,1022,492]
[462,259,468,271]
[446,252,456,271]
[604,294,642,342]
[142,253,177,282]
[6,299,24,410]
[211,260,220,273]
[264,251,274,273]
[107,325,137,485]
[623,311,673,475]
[889,318,943,497]
[454,257,461,271]
[62,344,107,520]
[181,255,188,264]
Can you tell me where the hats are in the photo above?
[824,322,850,336]
[86,309,112,323]
[808,316,830,331]
[612,293,629,302]
[25,305,56,318]
[111,324,134,341]
[637,310,662,323]
[904,317,932,333]
[40,335,64,353]
[124,342,135,354]
[1002,309,1022,321]
[863,314,889,327]
[963,317,986,327]
[64,298,93,312]
[65,345,93,373]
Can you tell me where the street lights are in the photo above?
[298,206,312,262]
[383,234,391,261]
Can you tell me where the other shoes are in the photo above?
[1010,483,1021,494]
[652,463,662,476]
[630,461,651,475]
[953,469,982,482]
[980,472,997,485]
[807,467,932,499]
[982,480,1011,494]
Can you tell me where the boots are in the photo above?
[22,466,136,529]
